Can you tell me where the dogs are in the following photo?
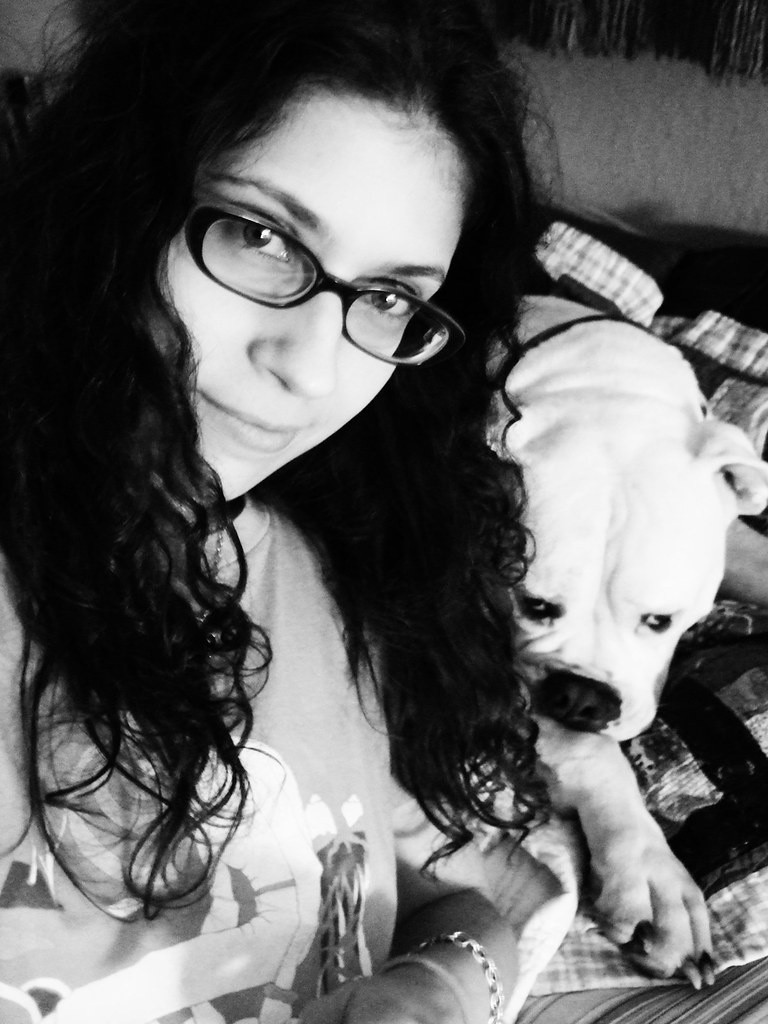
[486,294,768,993]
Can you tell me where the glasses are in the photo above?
[183,203,465,366]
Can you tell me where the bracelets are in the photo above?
[377,927,505,1024]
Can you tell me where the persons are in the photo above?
[0,0,573,1024]
